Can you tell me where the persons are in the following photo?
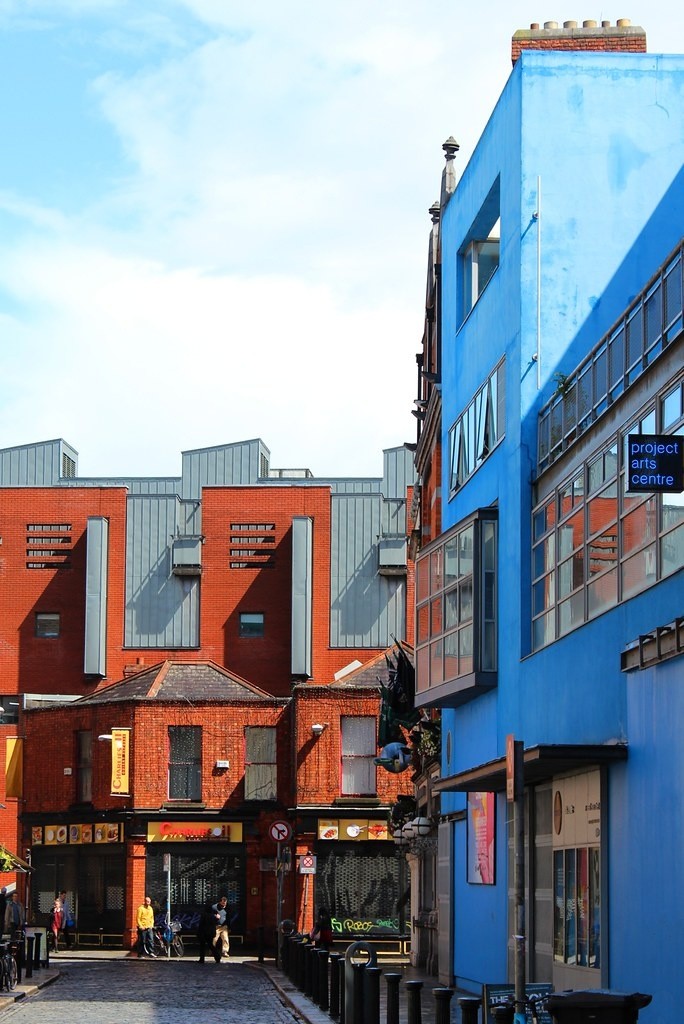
[136,896,158,958]
[4,893,25,935]
[47,891,74,953]
[211,896,229,957]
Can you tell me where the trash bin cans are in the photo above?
[543,989,652,1023]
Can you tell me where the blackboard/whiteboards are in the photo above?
[482,980,555,1024]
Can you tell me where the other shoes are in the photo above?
[150,953,158,958]
[222,951,229,957]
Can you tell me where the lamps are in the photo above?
[312,725,323,736]
[392,815,434,864]
